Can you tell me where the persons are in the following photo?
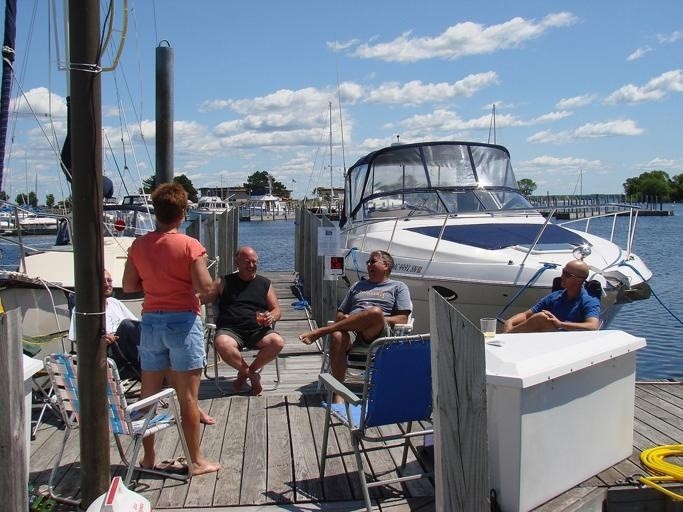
[200,245,285,397]
[503,259,601,333]
[298,248,412,404]
[68,268,216,425]
[121,183,222,476]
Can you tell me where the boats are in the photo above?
[0,181,288,237]
[18,205,179,298]
[320,103,658,334]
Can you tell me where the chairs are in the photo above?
[202,300,282,398]
[31,371,64,440]
[43,352,194,504]
[67,292,172,409]
[314,333,436,512]
[317,301,412,387]
[552,278,602,302]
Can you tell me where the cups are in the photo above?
[479,317,497,339]
[255,310,268,327]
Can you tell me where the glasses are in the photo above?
[562,269,585,280]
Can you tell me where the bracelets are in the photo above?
[272,316,275,323]
